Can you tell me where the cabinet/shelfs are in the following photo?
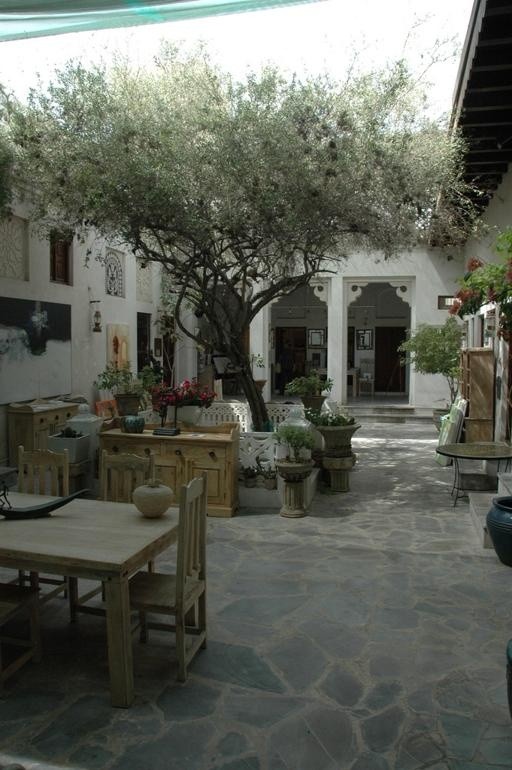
[460,347,496,443]
[7,402,77,473]
[98,422,241,519]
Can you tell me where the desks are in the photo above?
[358,378,375,397]
[0,490,177,709]
[315,368,359,397]
[435,443,511,506]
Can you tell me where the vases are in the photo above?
[167,404,202,424]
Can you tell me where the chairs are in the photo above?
[124,470,209,683]
[2,581,46,682]
[68,449,154,627]
[6,445,70,605]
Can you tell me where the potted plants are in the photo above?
[272,373,361,519]
[93,357,157,415]
[46,426,90,463]
[132,478,175,517]
[396,314,468,432]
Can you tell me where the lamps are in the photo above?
[89,299,104,334]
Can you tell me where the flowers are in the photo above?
[153,377,215,413]
